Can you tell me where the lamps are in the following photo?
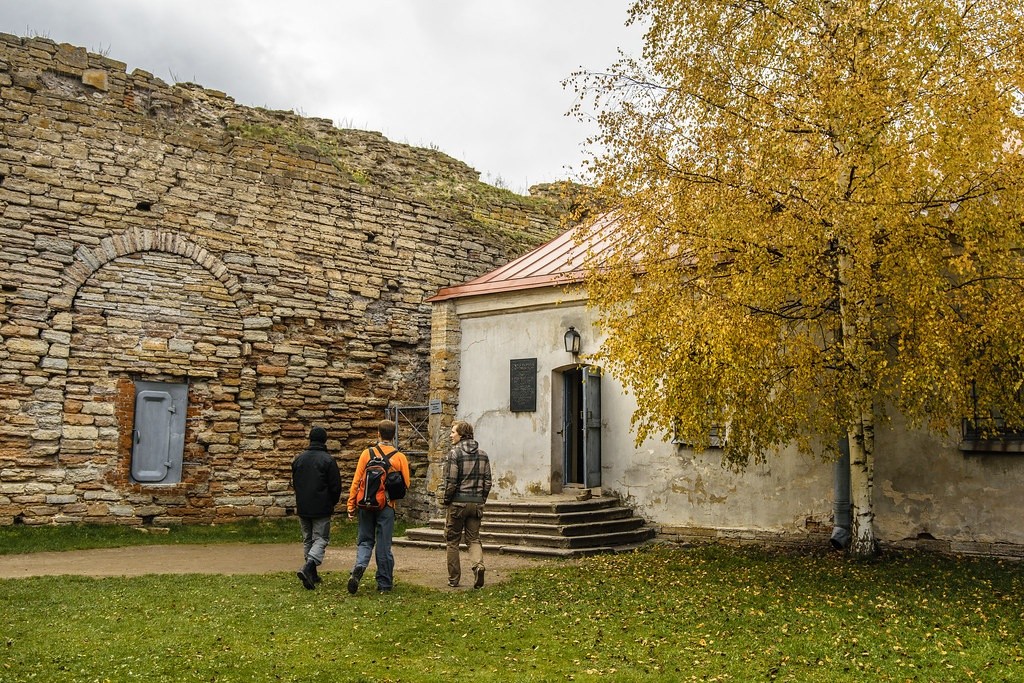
[564,325,584,359]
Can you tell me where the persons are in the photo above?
[291,427,342,589]
[441,420,492,589]
[347,420,410,593]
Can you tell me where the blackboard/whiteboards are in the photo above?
[509,357,538,412]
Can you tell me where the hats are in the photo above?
[310,427,327,444]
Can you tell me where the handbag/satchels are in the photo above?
[385,471,407,500]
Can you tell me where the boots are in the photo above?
[306,560,321,582]
[297,560,315,590]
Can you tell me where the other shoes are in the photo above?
[474,567,484,589]
[448,582,458,586]
[347,566,363,594]
[378,585,392,591]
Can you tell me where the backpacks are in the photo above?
[355,447,399,510]
